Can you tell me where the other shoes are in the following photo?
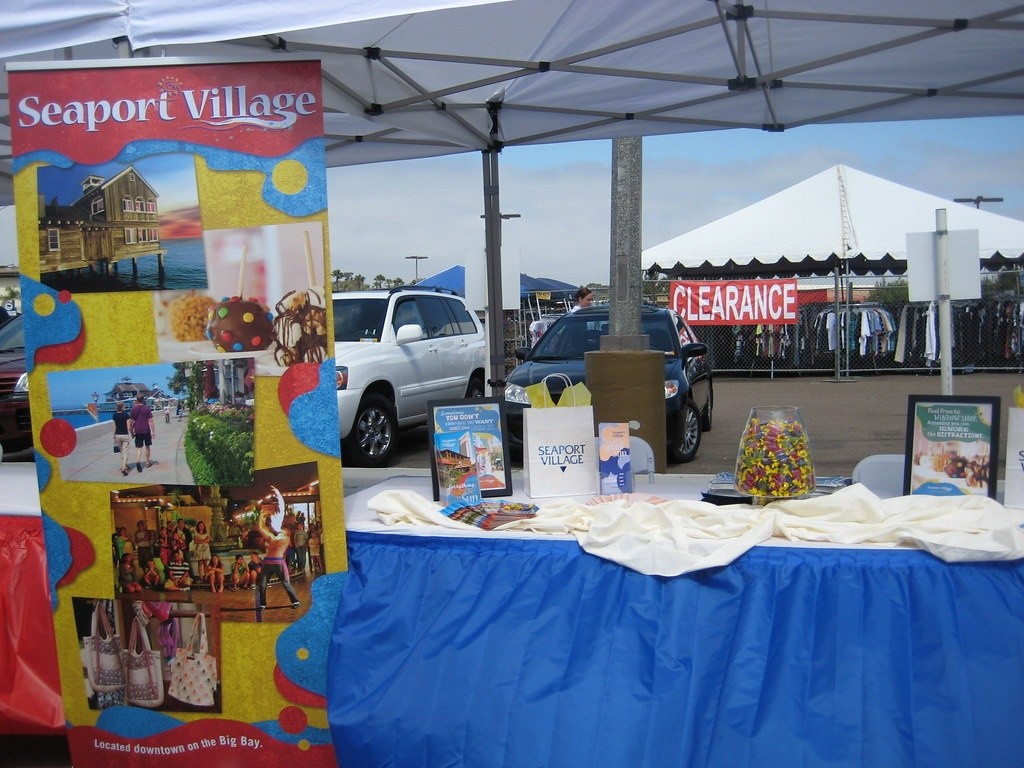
[244,587,250,590]
[180,587,190,591]
[233,588,236,591]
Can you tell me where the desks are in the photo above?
[325,475,1024,768]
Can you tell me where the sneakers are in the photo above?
[145,463,152,468]
[291,602,300,609]
[261,606,266,611]
[136,463,142,472]
[121,469,128,476]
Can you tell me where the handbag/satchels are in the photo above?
[168,612,217,706]
[114,446,120,453]
[522,372,601,499]
[83,600,126,693]
[124,617,165,708]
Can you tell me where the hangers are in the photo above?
[821,302,885,312]
[906,296,1024,309]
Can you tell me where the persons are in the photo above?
[571,288,593,314]
[112,505,324,611]
[163,402,171,423]
[176,401,185,422]
[113,393,156,476]
